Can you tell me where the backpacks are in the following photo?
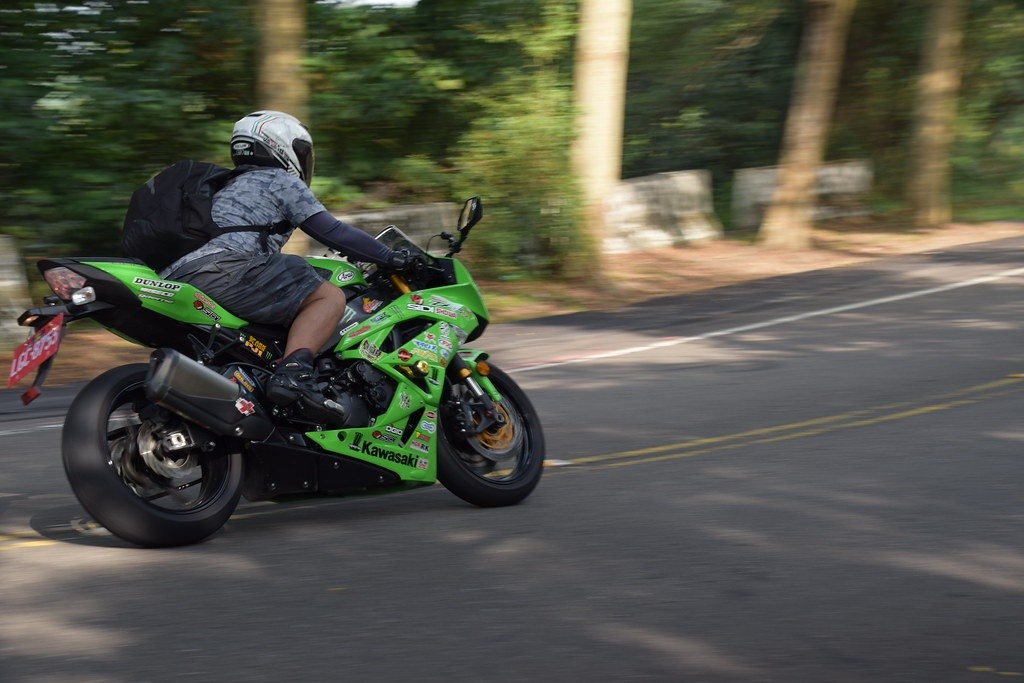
[121,160,296,272]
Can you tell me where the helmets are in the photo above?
[230,111,313,186]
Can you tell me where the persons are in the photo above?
[156,110,430,425]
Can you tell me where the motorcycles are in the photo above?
[7,196,546,547]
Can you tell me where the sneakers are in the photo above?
[264,350,345,417]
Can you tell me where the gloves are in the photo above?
[393,248,430,275]
